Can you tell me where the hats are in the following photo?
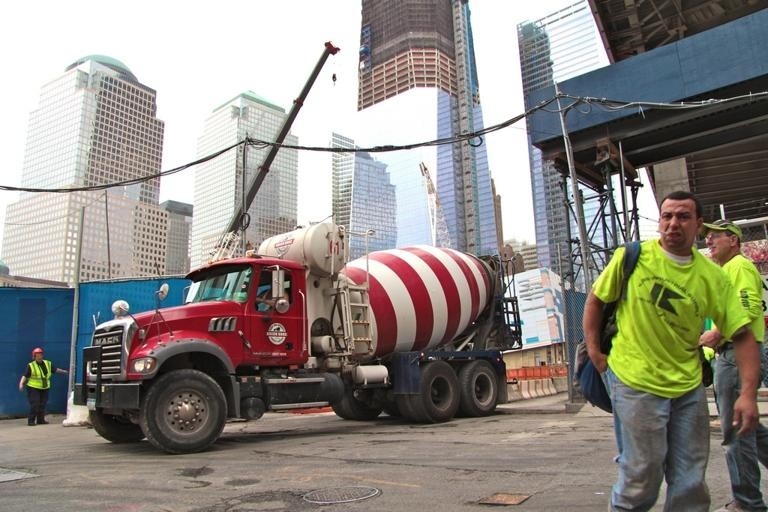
[702,218,743,239]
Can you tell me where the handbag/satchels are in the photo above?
[572,308,618,413]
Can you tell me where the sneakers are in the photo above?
[29,422,35,425]
[44,421,49,424]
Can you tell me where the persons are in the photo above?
[19,348,69,426]
[582,190,760,512]
[700,219,768,512]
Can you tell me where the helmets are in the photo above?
[32,347,44,359]
